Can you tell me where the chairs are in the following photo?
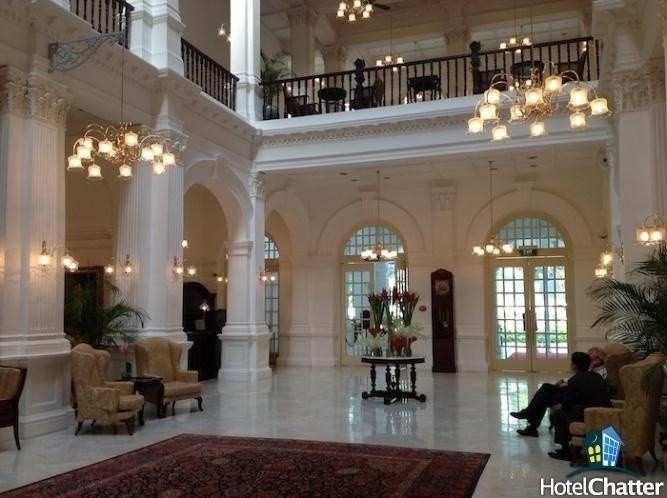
[135,337,204,416]
[553,51,587,83]
[569,352,666,476]
[348,77,384,111]
[549,348,634,430]
[0,365,27,450]
[283,84,320,117]
[479,67,508,94]
[71,343,144,436]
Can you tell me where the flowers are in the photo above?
[355,285,426,356]
[119,342,134,362]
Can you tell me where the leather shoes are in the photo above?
[548,448,572,460]
[510,409,527,419]
[517,426,538,437]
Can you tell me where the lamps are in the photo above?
[473,159,513,257]
[335,1,374,23]
[360,170,398,263]
[634,214,667,246]
[376,0,407,72]
[258,261,276,282]
[594,239,623,279]
[467,0,609,140]
[498,0,532,54]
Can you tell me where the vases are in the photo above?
[126,362,132,377]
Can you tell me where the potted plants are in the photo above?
[260,48,298,118]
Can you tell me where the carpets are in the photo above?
[0,433,491,497]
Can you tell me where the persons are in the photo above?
[511,347,608,437]
[548,352,615,462]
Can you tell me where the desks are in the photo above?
[407,74,439,103]
[510,60,545,86]
[317,87,347,114]
[361,352,426,405]
[114,377,164,426]
